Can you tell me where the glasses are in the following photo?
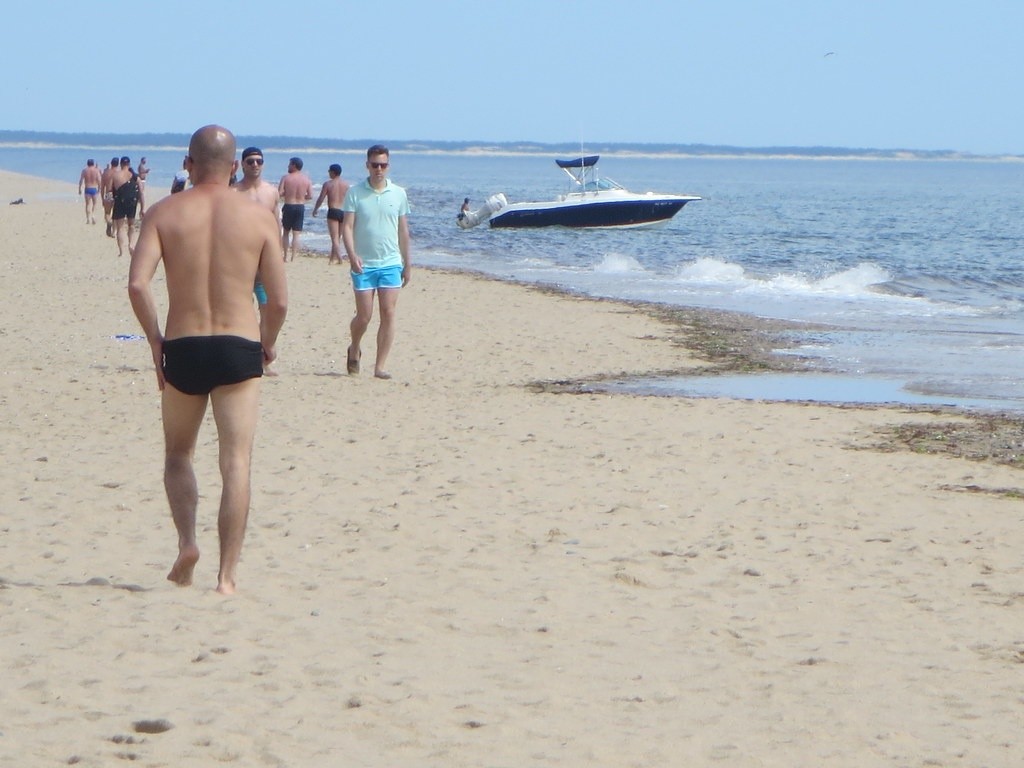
[368,161,388,169]
[245,159,264,165]
[328,170,332,172]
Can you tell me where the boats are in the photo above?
[454,156,704,232]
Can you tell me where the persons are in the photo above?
[170,157,191,196]
[313,164,350,266]
[129,126,288,596]
[138,157,150,190]
[460,197,470,212]
[343,145,410,378]
[78,160,101,224]
[100,158,119,238]
[279,158,313,262]
[95,162,103,194]
[108,156,145,256]
[229,148,282,376]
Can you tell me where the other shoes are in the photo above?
[347,346,361,374]
[375,369,391,379]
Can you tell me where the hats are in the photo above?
[242,147,263,160]
[112,158,119,163]
[120,157,130,163]
